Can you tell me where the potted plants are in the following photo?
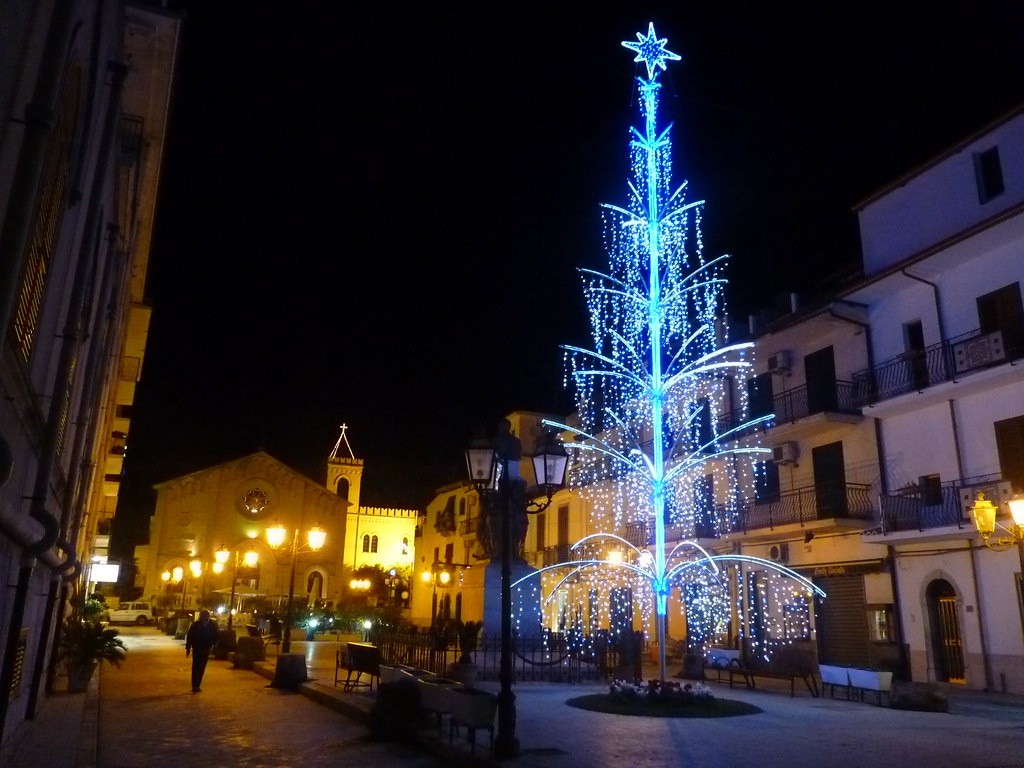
[447,620,485,690]
[271,595,368,642]
[48,612,127,692]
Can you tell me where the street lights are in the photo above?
[188,559,225,612]
[172,566,204,611]
[966,489,1024,587]
[264,517,327,654]
[215,544,260,632]
[464,417,570,758]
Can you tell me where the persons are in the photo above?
[313,594,324,613]
[185,610,219,692]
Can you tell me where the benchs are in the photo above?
[245,624,281,654]
[728,649,819,698]
[343,643,389,696]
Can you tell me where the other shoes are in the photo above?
[192,688,201,692]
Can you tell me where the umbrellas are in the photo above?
[212,584,266,610]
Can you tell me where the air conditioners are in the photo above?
[773,443,795,464]
[767,542,786,562]
[768,350,790,374]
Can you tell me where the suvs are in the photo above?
[107,601,156,626]
